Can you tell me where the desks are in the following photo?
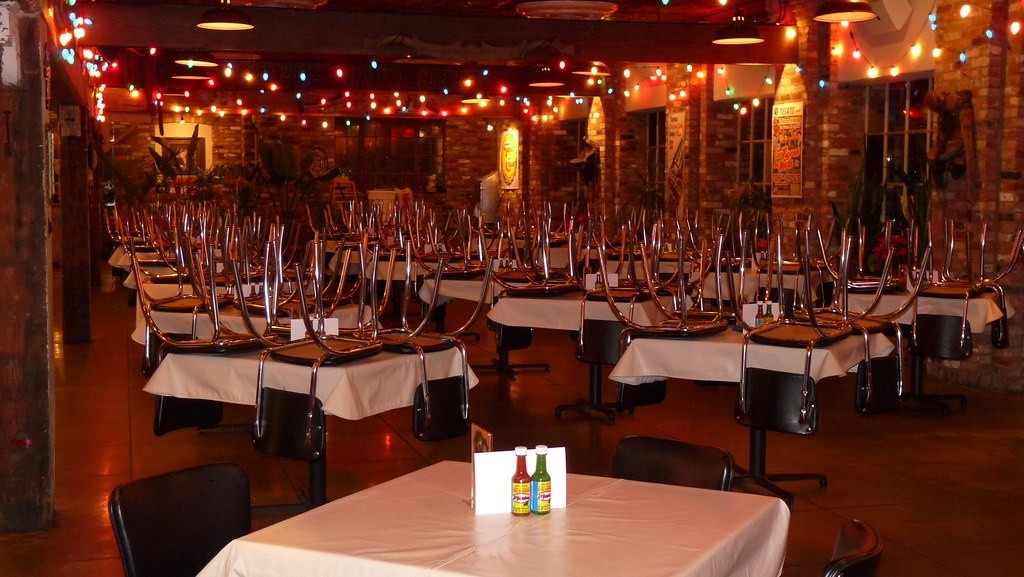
[608,323,895,508]
[364,262,485,341]
[533,245,575,268]
[836,283,1016,416]
[599,260,690,281]
[465,237,525,253]
[328,248,372,275]
[117,252,166,271]
[417,279,581,379]
[108,245,154,269]
[310,239,339,253]
[486,289,674,422]
[123,266,154,289]
[134,282,315,327]
[195,460,791,577]
[131,297,384,433]
[688,259,830,313]
[140,333,480,517]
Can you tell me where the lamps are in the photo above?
[524,0,878,87]
[163,0,255,97]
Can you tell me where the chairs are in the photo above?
[819,518,881,577]
[610,436,734,492]
[107,462,253,577]
[92,186,1024,461]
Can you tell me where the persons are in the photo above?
[262,149,288,189]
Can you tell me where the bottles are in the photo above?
[510,446,531,516]
[228,282,234,294]
[754,300,766,327]
[433,243,443,253]
[913,268,929,286]
[530,225,536,232]
[531,444,552,515]
[664,239,669,252]
[764,301,774,324]
[248,281,265,298]
[550,232,558,242]
[594,272,603,289]
[497,257,510,273]
[304,316,326,338]
[192,250,200,271]
[333,223,339,234]
